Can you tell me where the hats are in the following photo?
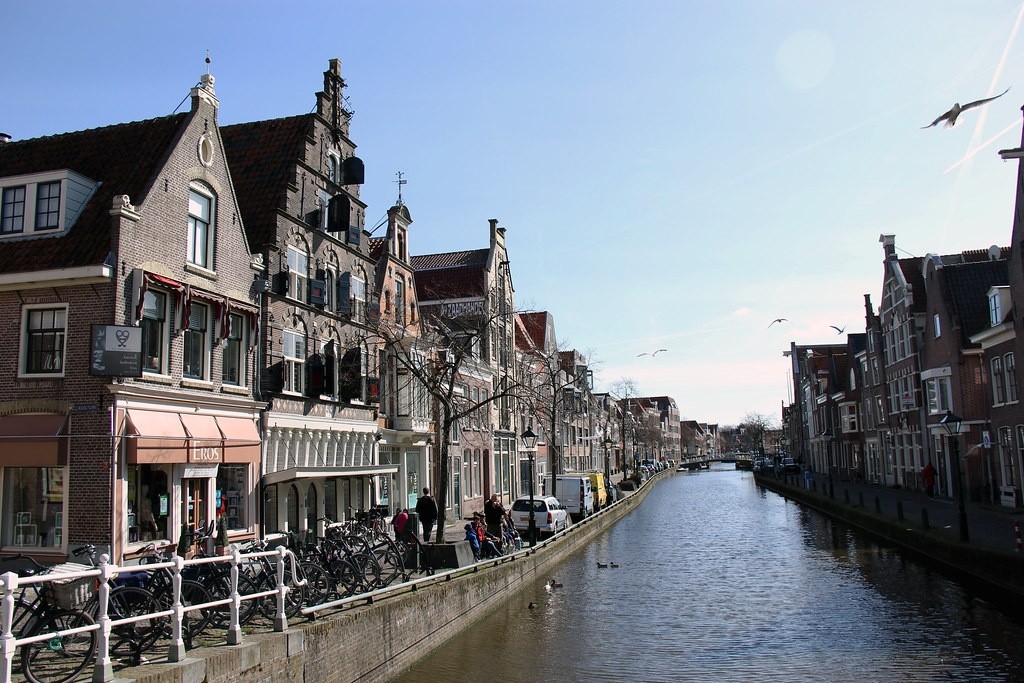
[464,524,472,530]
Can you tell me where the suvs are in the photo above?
[510,494,569,540]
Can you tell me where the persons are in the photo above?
[500,509,524,554]
[464,512,503,562]
[797,456,802,464]
[217,494,225,522]
[922,464,937,500]
[392,508,408,552]
[416,487,437,542]
[485,494,505,538]
[778,455,782,462]
[141,491,158,539]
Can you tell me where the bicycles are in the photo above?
[1,506,524,683]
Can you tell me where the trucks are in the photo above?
[564,471,609,512]
[542,474,598,523]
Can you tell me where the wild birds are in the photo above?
[542,580,563,591]
[528,602,534,609]
[768,318,794,328]
[829,325,847,336]
[595,562,607,568]
[637,349,667,358]
[921,86,1010,129]
[609,562,618,568]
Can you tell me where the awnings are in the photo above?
[0,415,68,467]
[125,410,261,461]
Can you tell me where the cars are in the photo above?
[637,457,676,482]
[752,450,802,475]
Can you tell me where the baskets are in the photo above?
[40,563,98,610]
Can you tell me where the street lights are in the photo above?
[603,436,614,506]
[519,423,540,546]
[780,436,787,484]
[821,428,834,498]
[632,441,639,488]
[939,407,970,542]
[663,446,666,469]
